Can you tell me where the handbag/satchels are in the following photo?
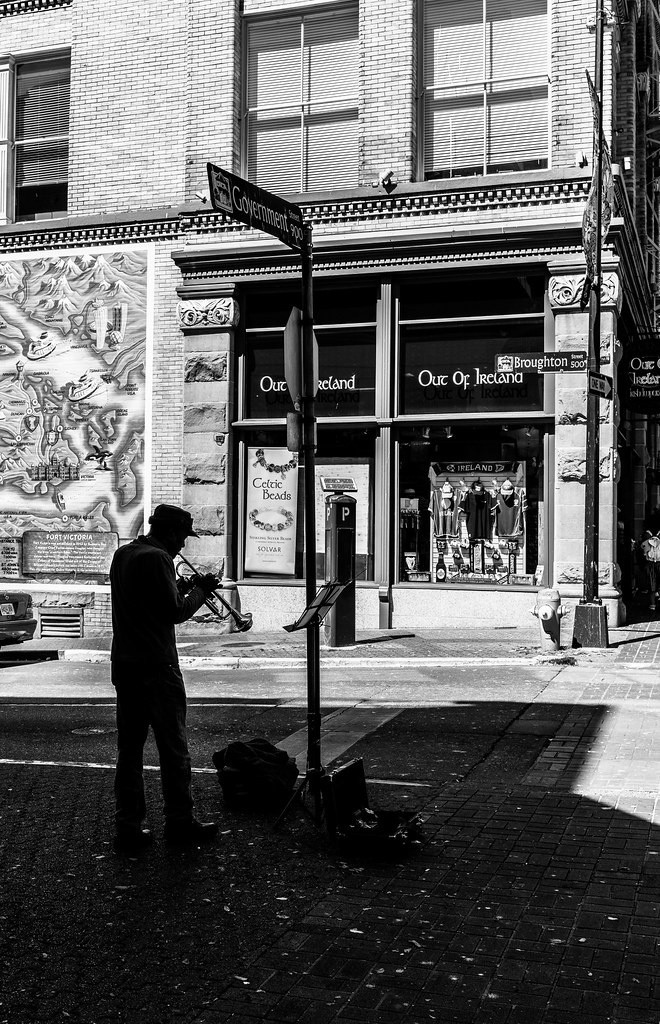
[213,739,300,804]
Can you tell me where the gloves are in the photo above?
[190,573,218,592]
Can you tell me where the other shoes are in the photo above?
[642,590,648,593]
[649,605,655,610]
[655,592,660,600]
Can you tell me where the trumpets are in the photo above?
[172,551,254,634]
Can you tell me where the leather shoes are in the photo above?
[163,820,217,844]
[118,828,153,855]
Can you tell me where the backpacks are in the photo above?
[641,530,660,563]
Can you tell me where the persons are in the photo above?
[108,506,224,853]
[641,505,660,611]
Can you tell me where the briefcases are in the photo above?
[320,757,430,864]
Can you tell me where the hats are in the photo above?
[501,480,513,495]
[471,481,483,495]
[442,484,454,498]
[149,504,200,538]
